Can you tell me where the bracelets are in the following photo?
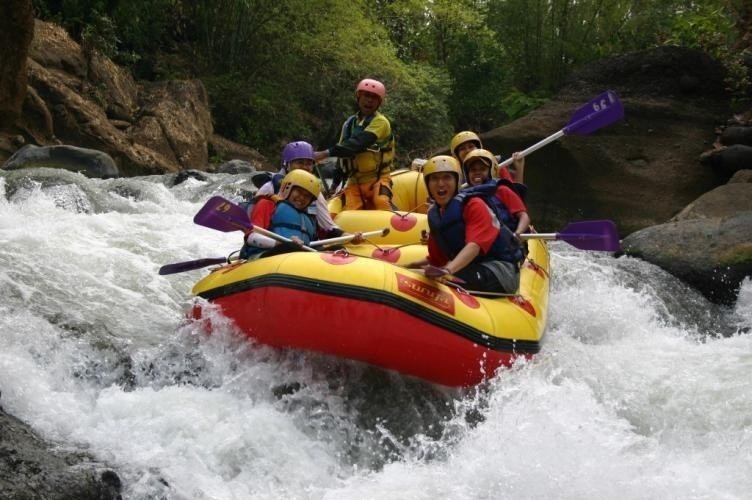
[441,267,451,275]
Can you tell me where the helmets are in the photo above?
[283,141,316,162]
[450,131,482,156]
[422,155,463,185]
[280,169,320,200]
[463,149,498,182]
[356,78,386,101]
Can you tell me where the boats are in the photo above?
[189,158,553,388]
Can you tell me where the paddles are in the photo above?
[427,88,624,204]
[159,229,389,275]
[193,196,317,251]
[420,265,448,278]
[421,220,621,252]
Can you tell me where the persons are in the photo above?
[420,148,531,257]
[257,141,366,245]
[445,130,526,184]
[243,168,323,263]
[312,78,398,211]
[424,155,521,298]
